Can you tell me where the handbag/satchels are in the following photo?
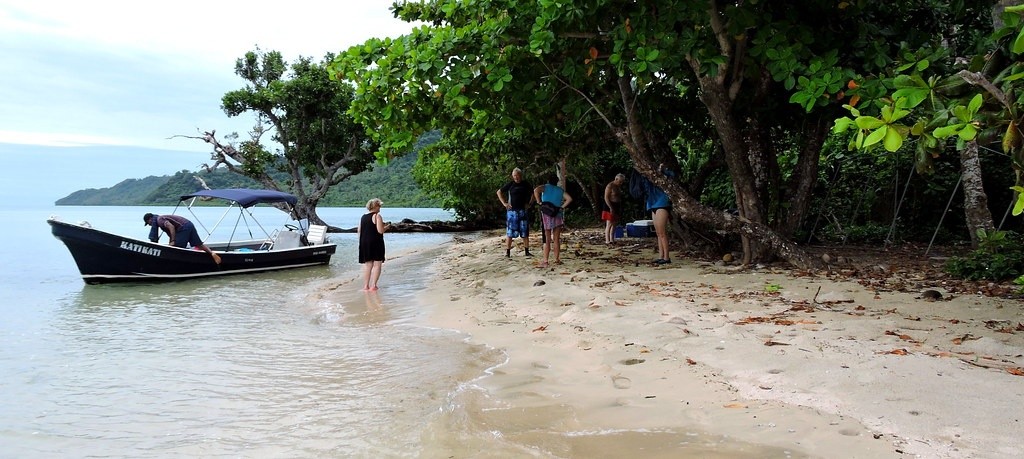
[541,202,562,216]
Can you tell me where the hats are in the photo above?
[144,213,153,226]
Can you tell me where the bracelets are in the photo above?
[170,239,174,243]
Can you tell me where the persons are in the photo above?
[644,165,679,265]
[602,173,625,244]
[497,167,534,258]
[357,198,391,290]
[534,176,572,265]
[143,213,221,264]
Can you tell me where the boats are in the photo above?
[46,189,338,285]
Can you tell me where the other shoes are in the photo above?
[506,255,512,260]
[606,241,611,243]
[553,260,564,265]
[210,252,221,264]
[611,241,617,244]
[650,258,663,263]
[656,258,671,265]
[526,253,533,256]
[534,262,547,267]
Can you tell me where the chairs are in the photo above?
[307,225,328,246]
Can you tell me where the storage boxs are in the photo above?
[626,220,656,238]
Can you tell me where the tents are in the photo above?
[159,188,310,251]
